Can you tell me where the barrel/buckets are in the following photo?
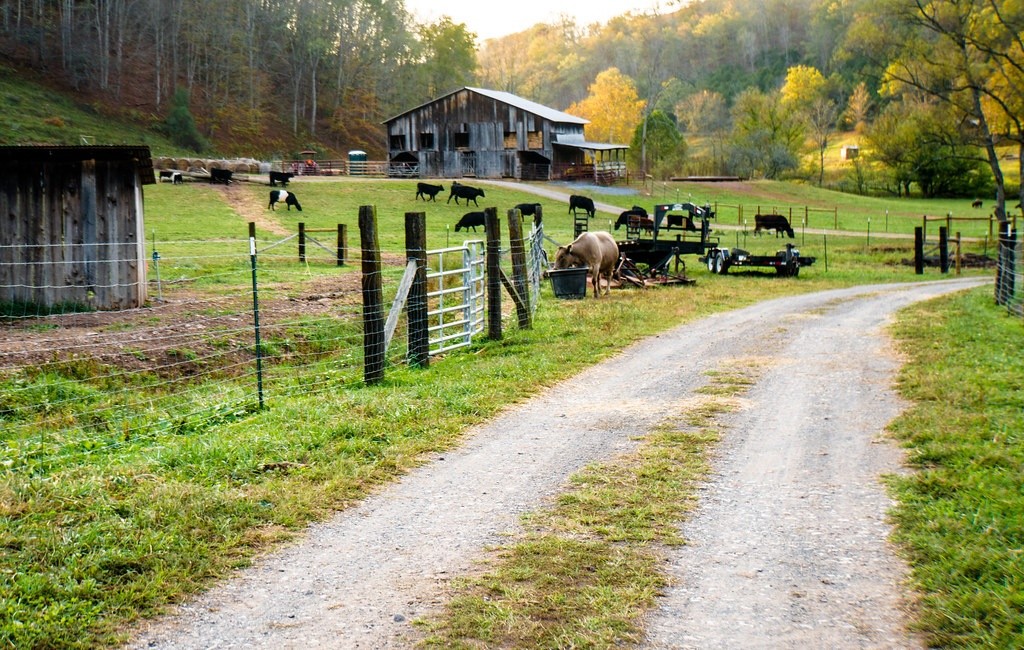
[159,158,263,174]
[546,268,589,300]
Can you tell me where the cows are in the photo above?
[754,213,795,239]
[454,210,487,233]
[515,202,542,223]
[972,200,983,209]
[550,233,620,299]
[447,180,486,207]
[614,204,655,237]
[268,170,295,188]
[416,183,445,204]
[567,195,597,219]
[209,167,234,186]
[267,190,303,212]
[666,213,697,234]
[160,171,185,186]
